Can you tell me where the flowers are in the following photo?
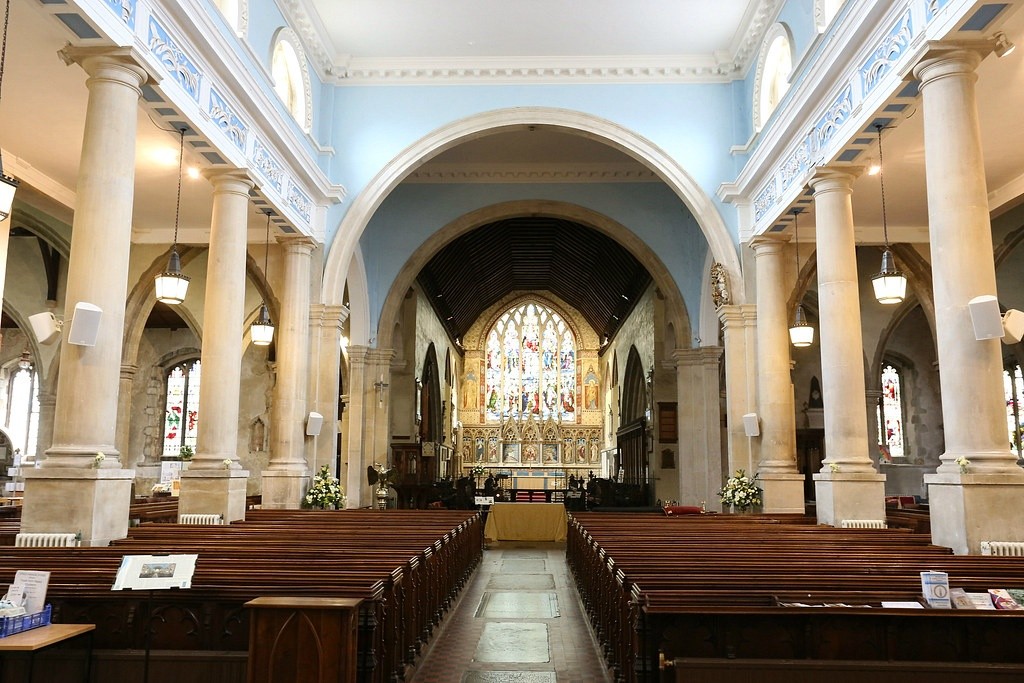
[176,445,192,461]
[829,464,840,474]
[720,468,765,509]
[956,456,970,476]
[307,465,346,509]
[222,459,232,472]
[92,453,106,468]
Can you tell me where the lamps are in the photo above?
[863,160,879,176]
[788,213,815,346]
[993,32,1015,57]
[250,211,275,345]
[871,125,909,305]
[29,312,65,344]
[155,127,190,305]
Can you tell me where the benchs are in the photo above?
[564,506,1024,683]
[0,508,491,683]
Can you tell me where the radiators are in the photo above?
[843,519,888,529]
[982,542,1024,557]
[179,514,224,526]
[15,533,81,547]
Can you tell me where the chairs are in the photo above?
[885,495,916,510]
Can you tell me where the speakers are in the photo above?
[969,294,1005,340]
[1002,309,1024,344]
[743,412,760,437]
[68,302,103,346]
[306,412,323,435]
[28,312,61,345]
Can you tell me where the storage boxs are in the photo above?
[0,605,52,639]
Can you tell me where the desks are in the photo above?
[0,624,98,683]
[485,502,567,542]
[243,595,364,683]
